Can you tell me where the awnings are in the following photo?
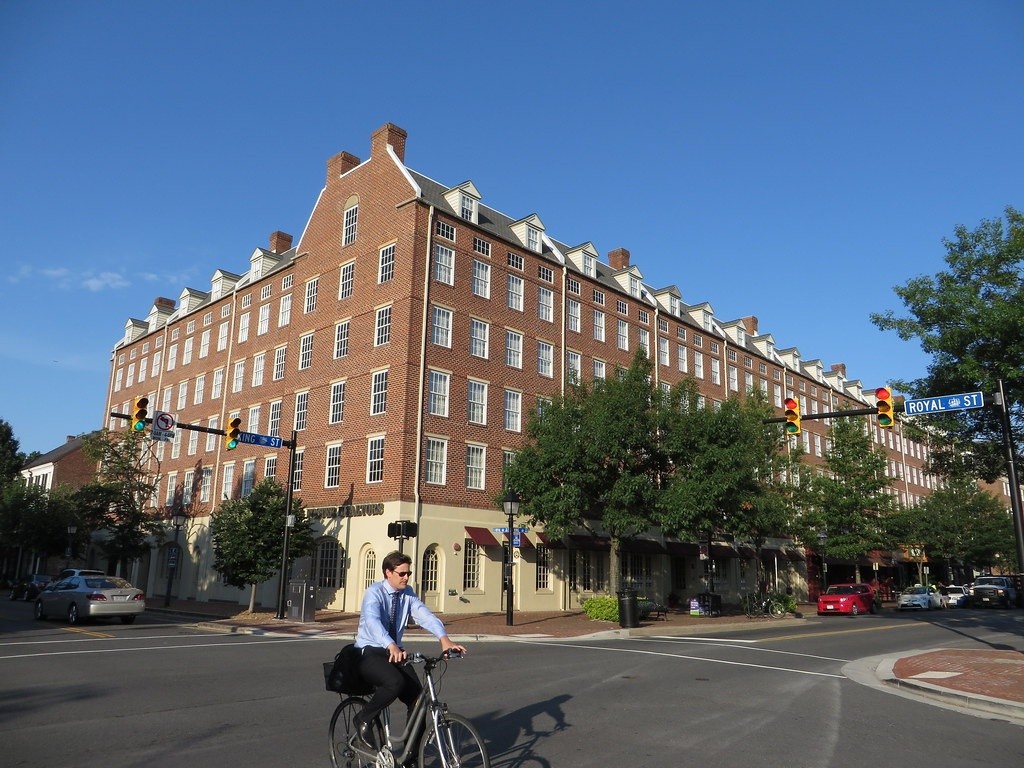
[564,517,806,561]
[465,526,565,548]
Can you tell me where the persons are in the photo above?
[352,551,466,768]
[939,583,949,608]
[964,589,970,594]
[845,576,853,583]
[6,579,13,595]
[758,577,769,593]
[871,575,896,591]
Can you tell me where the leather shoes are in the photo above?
[353,713,376,749]
[402,751,418,768]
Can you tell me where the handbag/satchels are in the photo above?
[328,643,370,695]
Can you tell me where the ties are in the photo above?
[388,591,400,656]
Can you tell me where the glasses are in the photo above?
[392,570,412,577]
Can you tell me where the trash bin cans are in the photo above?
[615,591,640,628]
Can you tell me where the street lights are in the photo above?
[163,507,188,607]
[66,518,79,569]
[503,486,522,622]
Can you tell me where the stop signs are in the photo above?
[132,396,149,432]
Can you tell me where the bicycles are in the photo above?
[743,591,786,619]
[328,646,492,768]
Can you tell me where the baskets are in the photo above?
[323,661,376,694]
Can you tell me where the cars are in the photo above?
[43,569,105,589]
[944,576,1023,610]
[8,575,51,603]
[897,586,945,610]
[34,575,146,626]
[816,583,878,616]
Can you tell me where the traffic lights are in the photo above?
[402,522,418,537]
[225,417,242,451]
[783,397,801,435]
[874,384,895,428]
